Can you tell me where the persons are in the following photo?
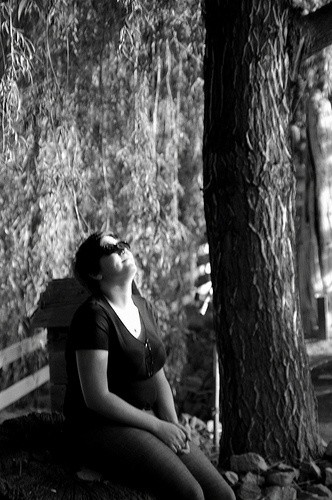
[70,230,236,500]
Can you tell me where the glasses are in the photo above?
[100,241,131,257]
